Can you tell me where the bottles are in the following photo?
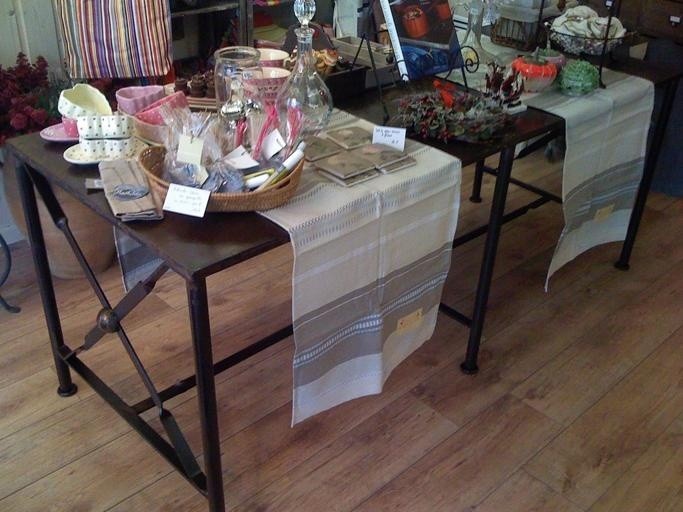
[275,0,333,148]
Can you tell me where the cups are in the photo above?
[211,45,292,132]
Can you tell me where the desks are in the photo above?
[7,45,681,512]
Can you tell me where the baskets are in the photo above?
[543,15,636,55]
[138,146,305,213]
[491,17,540,52]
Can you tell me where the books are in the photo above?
[378,0,466,83]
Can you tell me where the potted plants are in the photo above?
[510,48,601,95]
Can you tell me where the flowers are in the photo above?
[1,52,60,140]
